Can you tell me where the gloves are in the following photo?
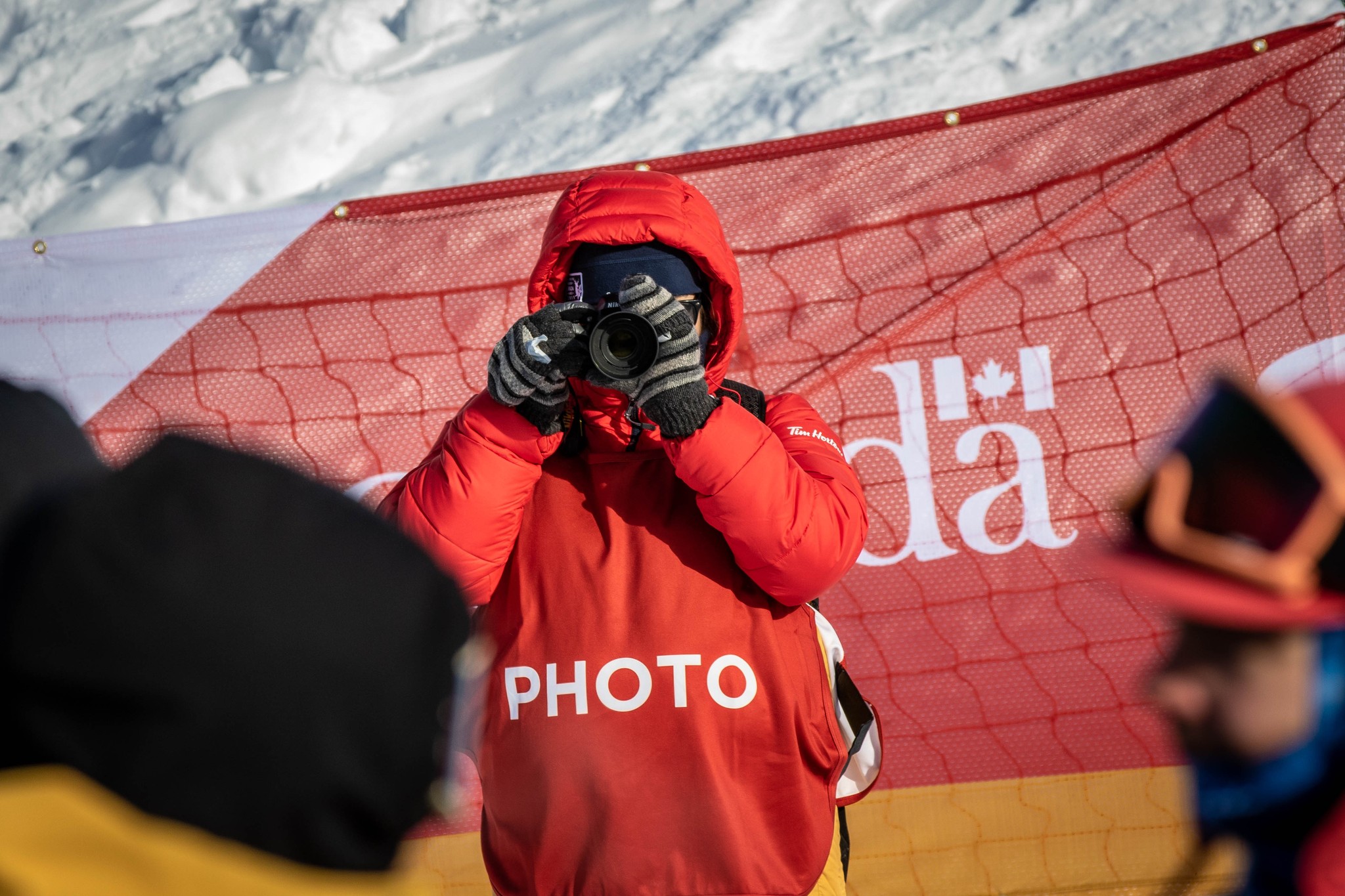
[487,302,589,406]
[586,273,721,438]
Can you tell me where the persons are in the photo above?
[0,383,487,896]
[1087,370,1345,896]
[372,172,867,895]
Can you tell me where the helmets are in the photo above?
[1091,333,1345,628]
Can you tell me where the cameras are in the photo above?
[559,307,659,382]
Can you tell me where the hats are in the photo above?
[565,243,705,307]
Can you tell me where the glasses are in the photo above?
[675,299,701,326]
[1107,369,1345,602]
